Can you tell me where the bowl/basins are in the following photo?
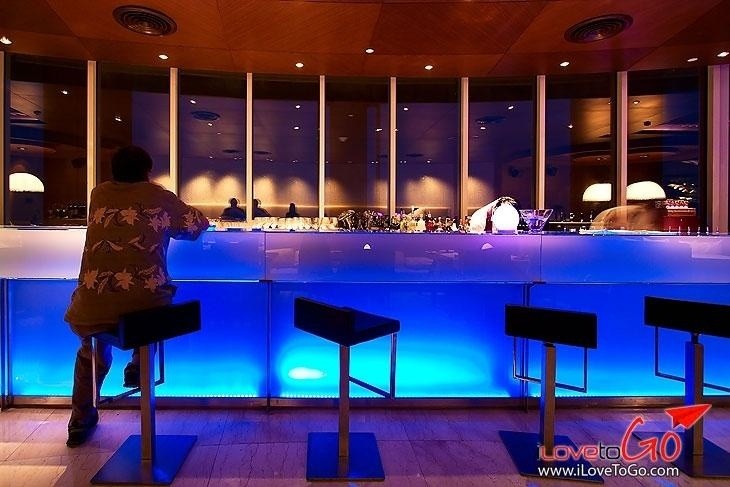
[519,209,554,232]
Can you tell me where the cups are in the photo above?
[247,217,308,231]
[304,218,311,230]
[313,218,319,230]
[321,217,330,231]
[217,221,246,228]
[570,213,575,223]
[330,217,338,228]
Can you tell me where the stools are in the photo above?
[632,294,729,477]
[499,304,604,484]
[295,297,400,482]
[90,299,201,485]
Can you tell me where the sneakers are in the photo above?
[65,411,100,447]
[123,362,140,388]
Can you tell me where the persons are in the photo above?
[220,198,244,218]
[253,199,270,217]
[286,203,300,217]
[65,146,209,448]
